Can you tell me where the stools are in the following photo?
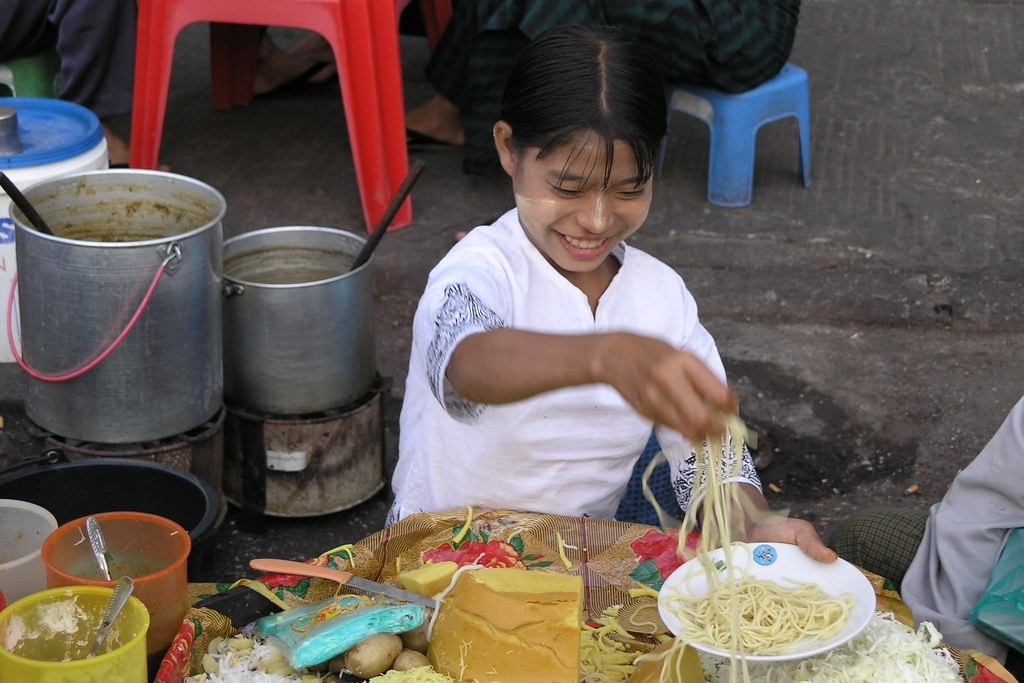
[129,0,452,235]
[654,62,813,207]
[0,50,60,99]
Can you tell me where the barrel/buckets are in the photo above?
[0,498,59,611]
[0,96,109,363]
[43,511,191,683]
[0,586,149,683]
[0,459,218,582]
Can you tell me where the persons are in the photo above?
[405,0,800,239]
[384,24,837,562]
[827,392,1024,668]
[0,1,340,175]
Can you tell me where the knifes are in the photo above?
[250,558,441,609]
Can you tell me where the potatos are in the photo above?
[204,636,321,683]
[306,619,433,683]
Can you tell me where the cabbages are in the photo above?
[767,609,966,683]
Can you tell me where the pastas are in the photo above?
[630,412,856,683]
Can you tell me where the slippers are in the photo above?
[403,128,463,153]
[254,61,340,102]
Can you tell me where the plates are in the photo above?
[658,541,878,662]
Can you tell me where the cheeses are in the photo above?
[424,564,583,683]
[398,560,460,598]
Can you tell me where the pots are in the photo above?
[223,226,379,416]
[9,169,227,444]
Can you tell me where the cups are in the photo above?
[0,106,24,156]
